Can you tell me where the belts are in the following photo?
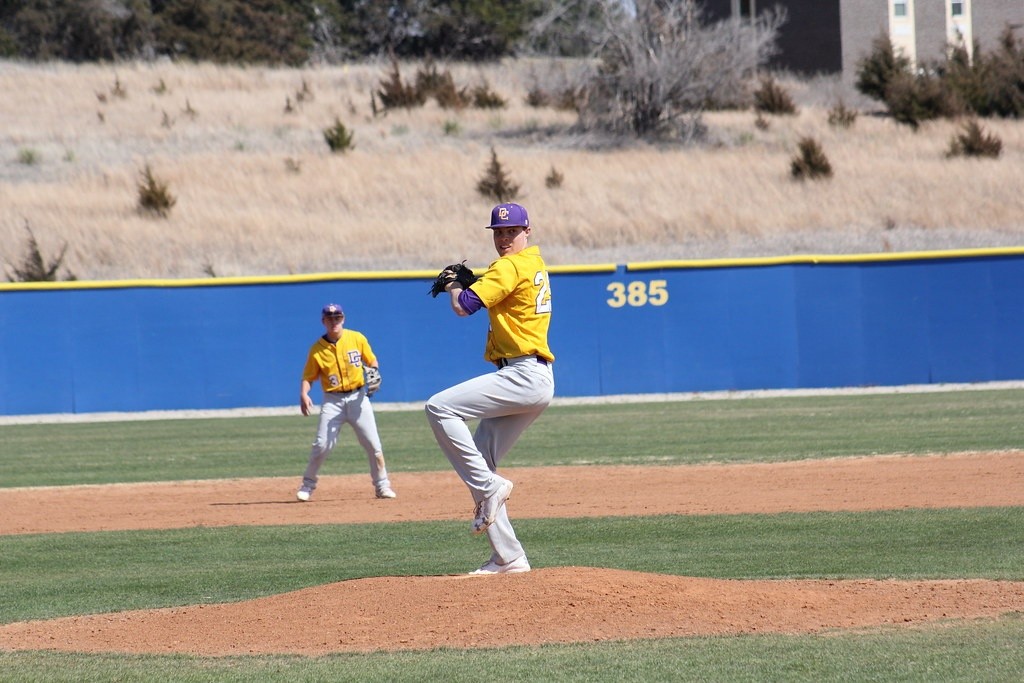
[325,386,361,394]
[498,355,547,370]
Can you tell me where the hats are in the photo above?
[322,303,343,320]
[485,202,529,227]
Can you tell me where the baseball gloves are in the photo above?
[431,264,477,298]
[362,365,382,397]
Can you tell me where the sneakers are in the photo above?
[376,488,396,498]
[296,484,312,501]
[468,554,530,575]
[472,480,513,535]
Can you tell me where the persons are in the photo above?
[425,202,556,575]
[297,304,396,501]
